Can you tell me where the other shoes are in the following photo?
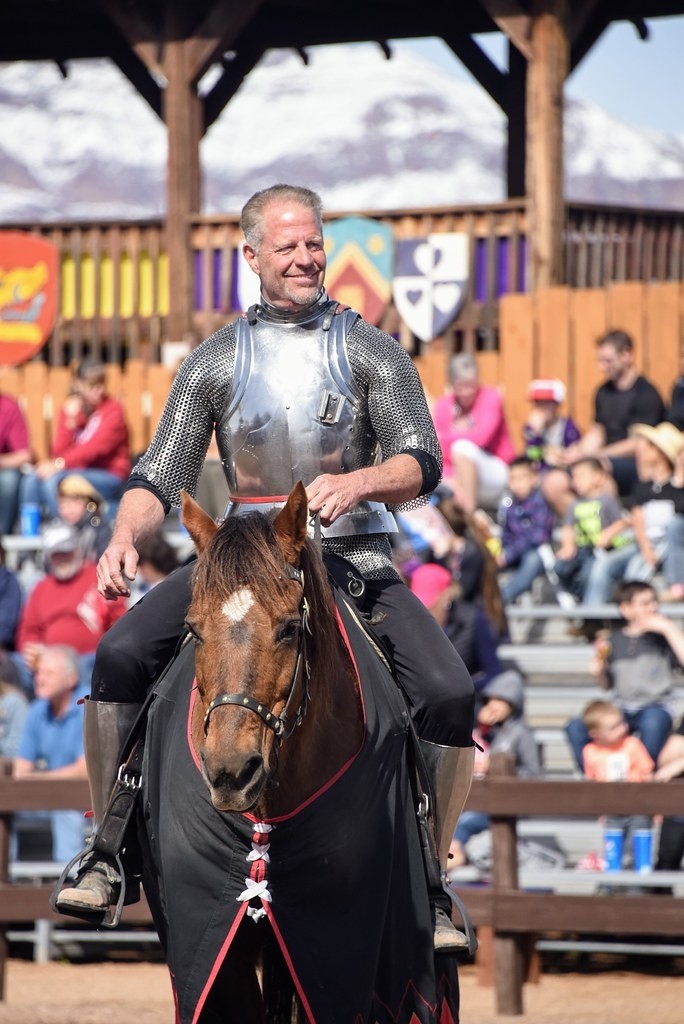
[433,907,468,952]
[56,871,141,915]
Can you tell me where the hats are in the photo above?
[628,422,684,466]
[524,378,565,404]
[44,525,77,558]
[409,564,452,611]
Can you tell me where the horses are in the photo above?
[126,479,450,1024]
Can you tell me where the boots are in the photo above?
[633,830,652,871]
[603,830,624,870]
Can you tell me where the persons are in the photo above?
[1,324,684,889]
[49,183,485,966]
